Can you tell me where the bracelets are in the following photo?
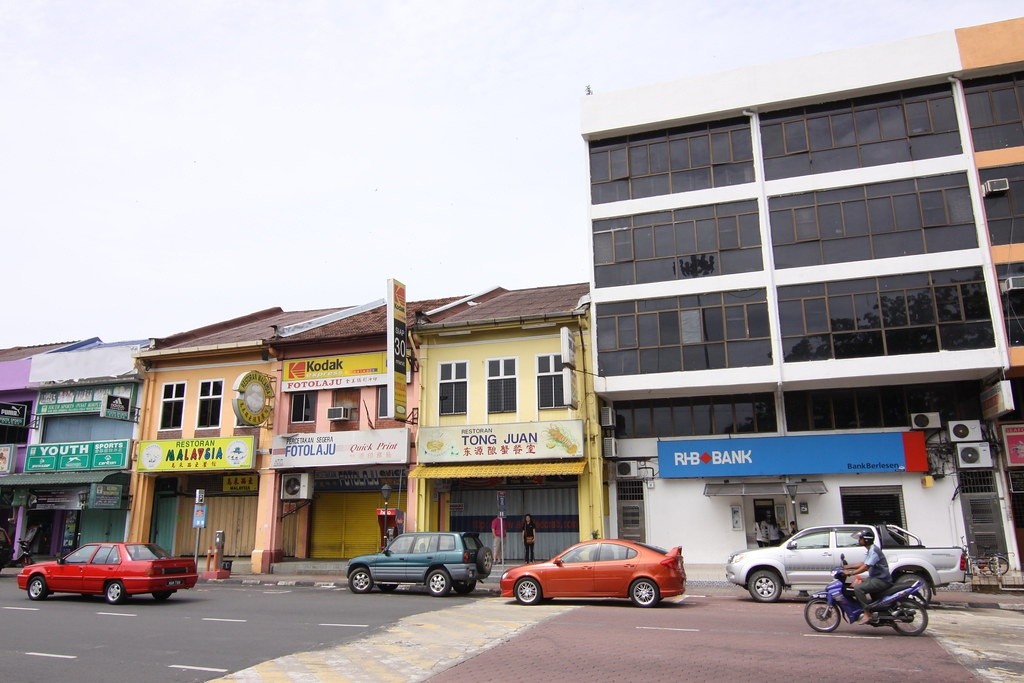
[849,571,853,576]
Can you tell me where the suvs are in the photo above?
[345,531,493,596]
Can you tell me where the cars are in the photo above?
[17,541,198,605]
[499,538,687,609]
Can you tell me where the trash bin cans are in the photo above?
[223,560,233,571]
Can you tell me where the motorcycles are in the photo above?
[803,553,932,636]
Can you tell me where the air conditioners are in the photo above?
[1005,276,1024,290]
[616,460,637,477]
[281,473,315,500]
[604,437,618,457]
[910,411,943,430]
[947,420,984,443]
[954,442,994,469]
[327,405,350,420]
[601,406,616,426]
[984,178,1010,193]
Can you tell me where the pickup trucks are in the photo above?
[726,522,969,604]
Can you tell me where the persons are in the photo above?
[786,521,798,539]
[754,512,781,548]
[491,508,507,565]
[522,514,536,563]
[842,528,892,625]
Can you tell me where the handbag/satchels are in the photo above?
[776,530,787,539]
[526,536,533,543]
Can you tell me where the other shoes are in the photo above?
[858,616,873,625]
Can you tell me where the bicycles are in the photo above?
[961,536,1010,575]
[18,538,32,567]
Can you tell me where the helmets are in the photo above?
[857,528,874,546]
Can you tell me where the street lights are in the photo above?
[380,482,392,551]
